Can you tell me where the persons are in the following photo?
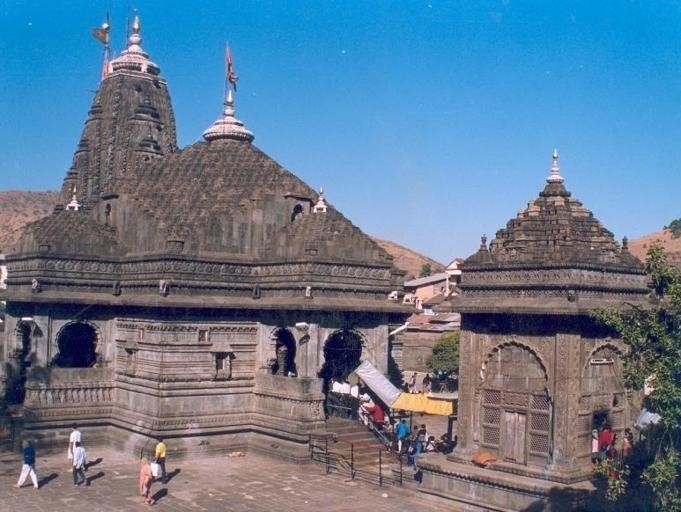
[153,435,169,483]
[71,440,89,487]
[138,456,158,506]
[592,423,634,467]
[66,421,83,472]
[326,371,454,471]
[12,441,42,489]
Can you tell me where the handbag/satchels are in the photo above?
[150,463,162,477]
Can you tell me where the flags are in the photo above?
[225,46,239,92]
